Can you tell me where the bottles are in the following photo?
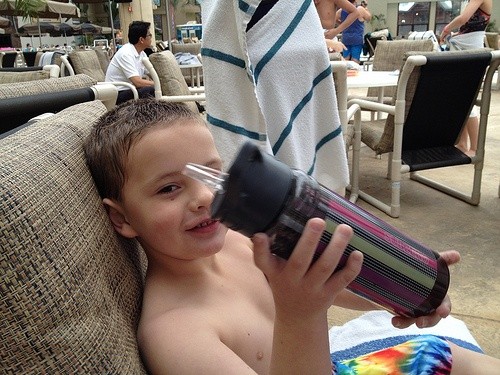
[182,141,450,319]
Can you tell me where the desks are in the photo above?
[180,64,202,87]
[347,69,399,121]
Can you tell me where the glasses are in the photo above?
[146,33,152,37]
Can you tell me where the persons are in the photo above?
[82,92,500,375]
[64,43,67,46]
[26,43,31,50]
[314,0,371,64]
[105,21,155,105]
[440,0,493,159]
[201,0,350,199]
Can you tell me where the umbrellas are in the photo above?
[0,0,133,54]
[154,26,163,35]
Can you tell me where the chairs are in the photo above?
[0,38,500,375]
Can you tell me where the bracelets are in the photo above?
[443,29,448,33]
